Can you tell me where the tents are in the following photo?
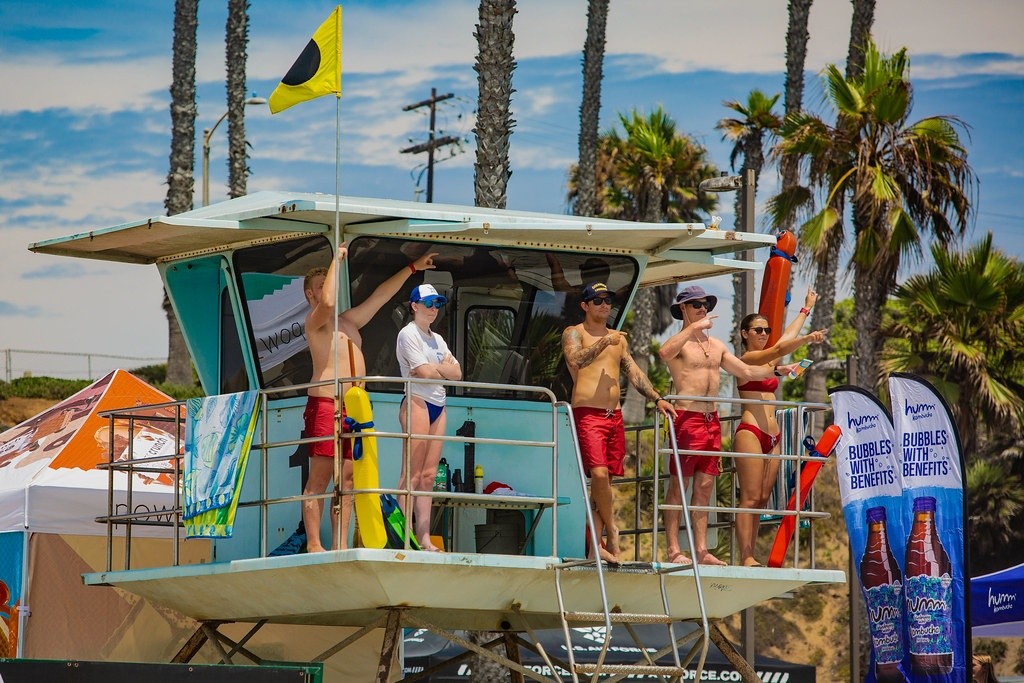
[1,369,405,683]
[970,563,1024,683]
[401,621,816,683]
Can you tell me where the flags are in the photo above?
[827,372,971,683]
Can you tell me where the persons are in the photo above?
[396,283,462,551]
[562,282,678,564]
[660,286,805,565]
[267,5,342,114]
[301,242,439,552]
[733,284,829,566]
[972,651,998,683]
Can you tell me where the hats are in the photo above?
[408,283,448,306]
[670,286,718,320]
[582,283,617,302]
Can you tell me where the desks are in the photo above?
[430,496,570,555]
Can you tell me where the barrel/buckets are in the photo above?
[474,522,519,555]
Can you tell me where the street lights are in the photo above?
[201,97,269,205]
[698,172,756,315]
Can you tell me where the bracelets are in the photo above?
[408,262,417,274]
[655,397,665,405]
[800,307,810,316]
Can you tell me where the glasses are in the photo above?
[748,326,772,335]
[591,297,613,305]
[689,301,712,310]
[420,299,442,309]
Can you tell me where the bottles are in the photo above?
[859,506,912,683]
[439,458,451,491]
[903,496,955,676]
[475,466,485,494]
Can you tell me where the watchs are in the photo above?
[774,366,783,376]
